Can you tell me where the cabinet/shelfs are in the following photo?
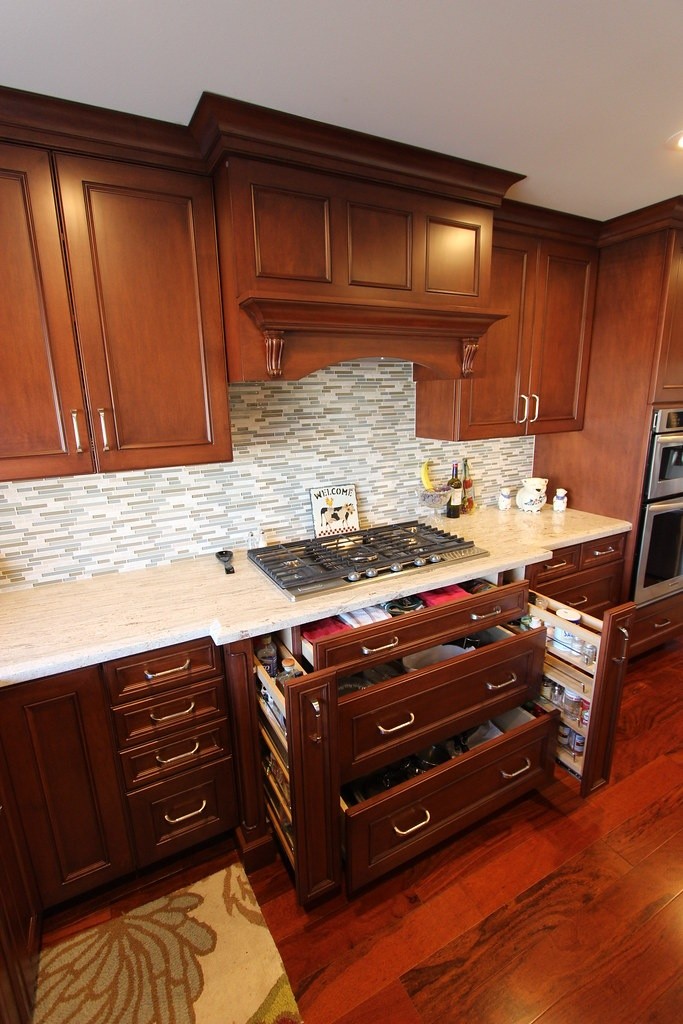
[0,86,600,482]
[0,534,631,1024]
[535,194,683,658]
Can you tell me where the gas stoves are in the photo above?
[247,519,490,602]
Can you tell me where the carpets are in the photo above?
[37,860,299,1024]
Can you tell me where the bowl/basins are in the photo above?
[403,644,468,673]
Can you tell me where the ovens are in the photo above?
[634,408,683,609]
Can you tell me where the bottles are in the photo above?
[257,635,278,677]
[520,597,597,756]
[247,532,257,550]
[276,658,299,697]
[447,461,462,518]
[460,458,476,516]
[258,531,267,548]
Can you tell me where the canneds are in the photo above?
[539,676,590,757]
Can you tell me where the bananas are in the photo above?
[421,458,435,493]
